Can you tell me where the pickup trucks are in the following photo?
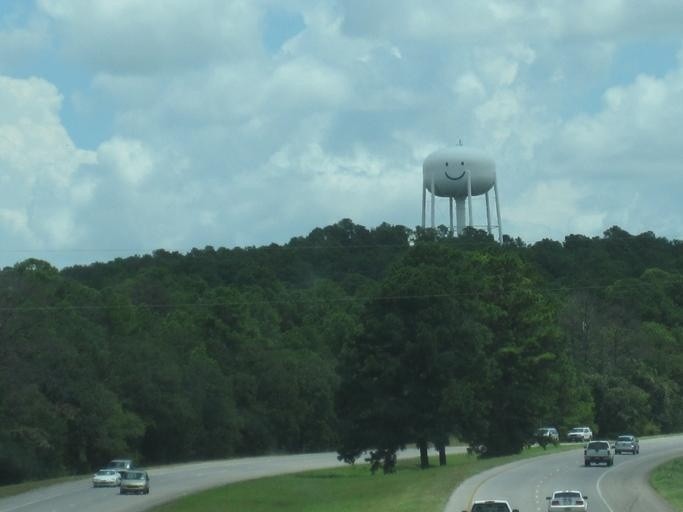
[567,427,592,443]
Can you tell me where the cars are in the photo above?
[615,435,639,455]
[546,490,588,512]
[533,428,559,441]
[92,459,150,494]
[461,500,519,512]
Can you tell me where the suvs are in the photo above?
[583,441,616,467]
